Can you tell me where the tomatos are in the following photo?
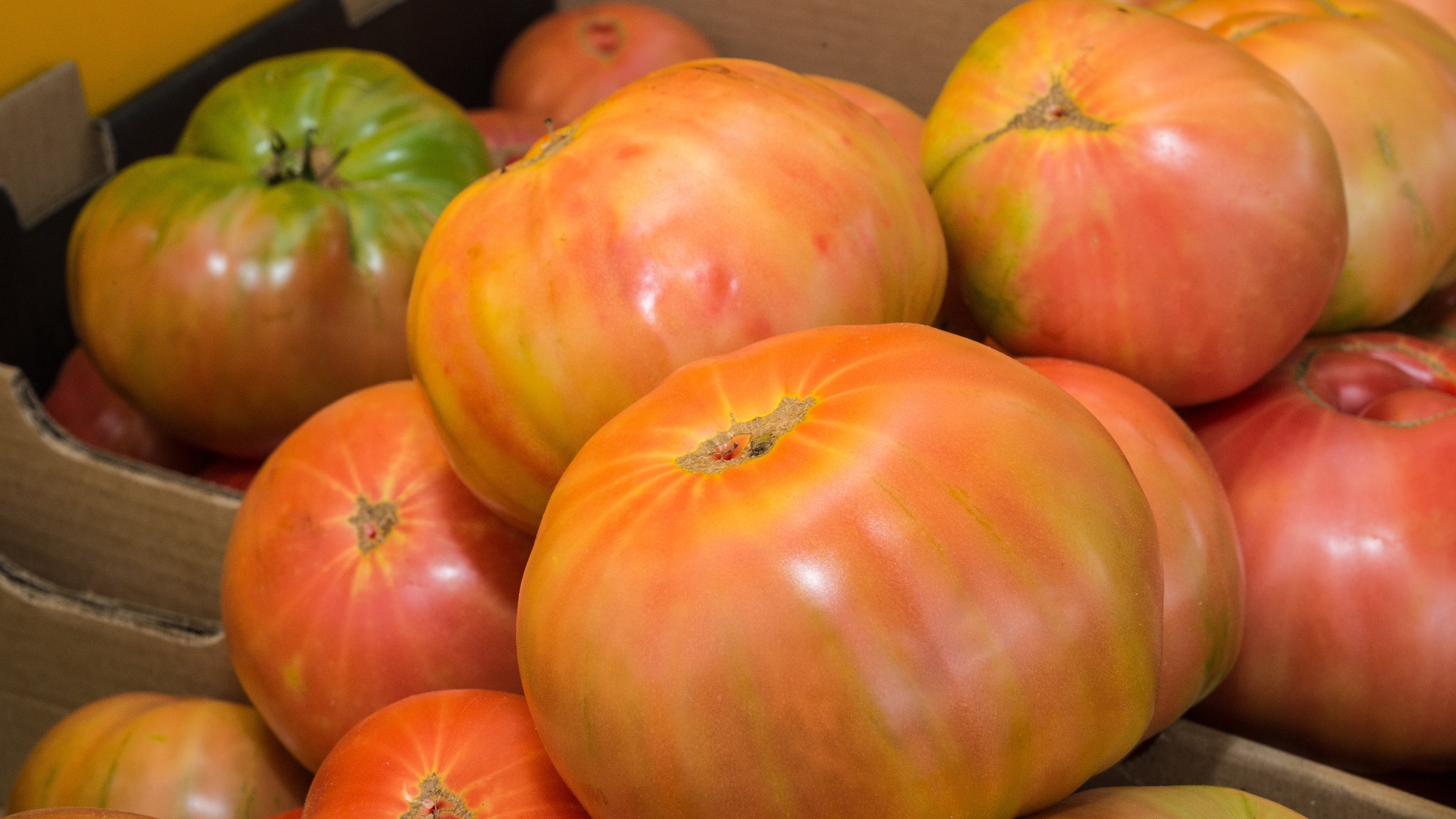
[1,0,1456,819]
